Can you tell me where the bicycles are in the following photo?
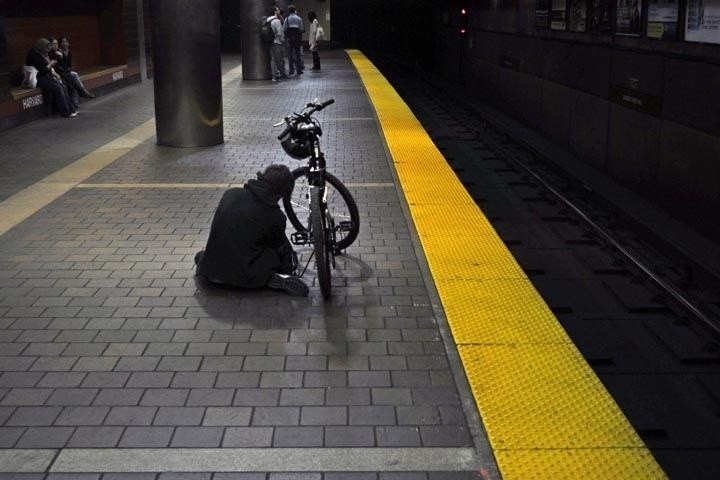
[273,97,360,301]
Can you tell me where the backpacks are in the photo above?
[261,25,274,45]
[315,27,324,41]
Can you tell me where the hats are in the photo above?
[258,163,294,197]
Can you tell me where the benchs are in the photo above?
[7,64,130,114]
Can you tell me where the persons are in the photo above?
[194,163,311,299]
[277,8,284,26]
[261,4,291,83]
[48,37,81,115]
[58,35,97,98]
[307,10,321,71]
[30,38,79,119]
[281,4,306,75]
[291,33,305,70]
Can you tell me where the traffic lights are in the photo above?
[458,7,470,36]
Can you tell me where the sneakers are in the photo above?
[309,65,320,70]
[289,66,305,74]
[82,92,95,99]
[61,102,81,119]
[271,76,289,81]
[267,273,311,298]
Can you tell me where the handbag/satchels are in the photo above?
[283,26,302,43]
